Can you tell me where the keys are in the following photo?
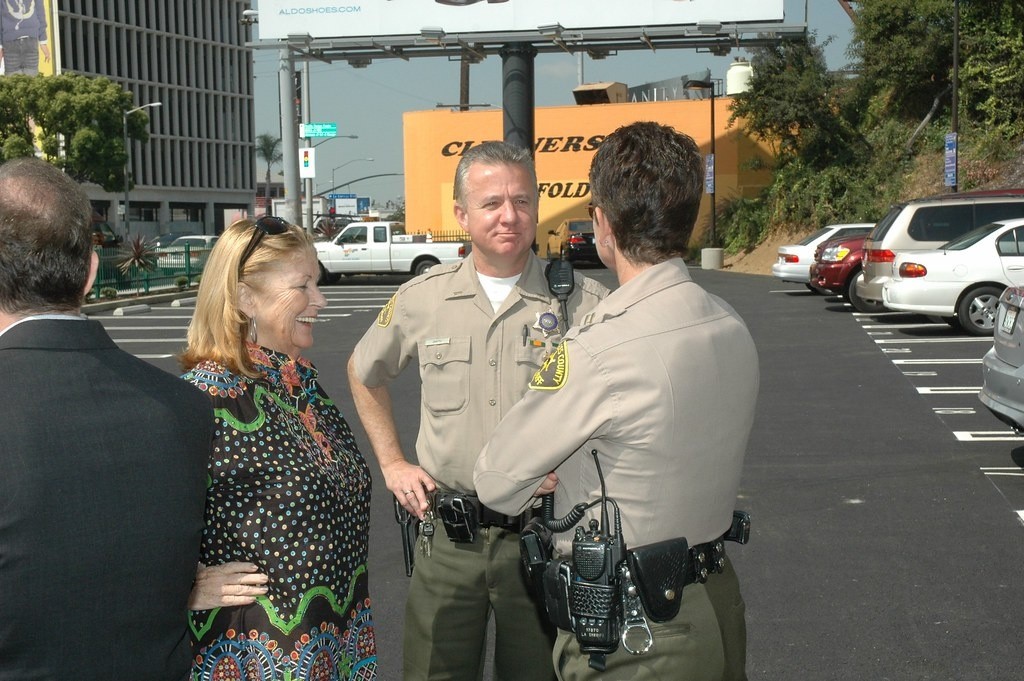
[418,514,435,557]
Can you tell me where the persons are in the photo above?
[471,122,759,680]
[0,156,214,681]
[177,213,379,681]
[347,141,613,681]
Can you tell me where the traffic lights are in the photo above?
[329,207,336,219]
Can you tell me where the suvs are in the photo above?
[808,188,1024,295]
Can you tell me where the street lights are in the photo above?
[331,158,374,199]
[681,80,718,248]
[122,102,162,241]
[303,135,359,198]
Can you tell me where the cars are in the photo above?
[157,235,220,275]
[977,284,1024,438]
[770,222,878,282]
[546,217,605,268]
[881,217,1024,336]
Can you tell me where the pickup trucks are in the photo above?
[313,221,472,285]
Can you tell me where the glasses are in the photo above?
[239,215,289,271]
[588,200,609,219]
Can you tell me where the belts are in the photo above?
[680,536,727,591]
[429,488,522,532]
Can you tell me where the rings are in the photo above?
[402,489,414,495]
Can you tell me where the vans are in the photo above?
[854,193,1024,301]
[90,206,132,289]
